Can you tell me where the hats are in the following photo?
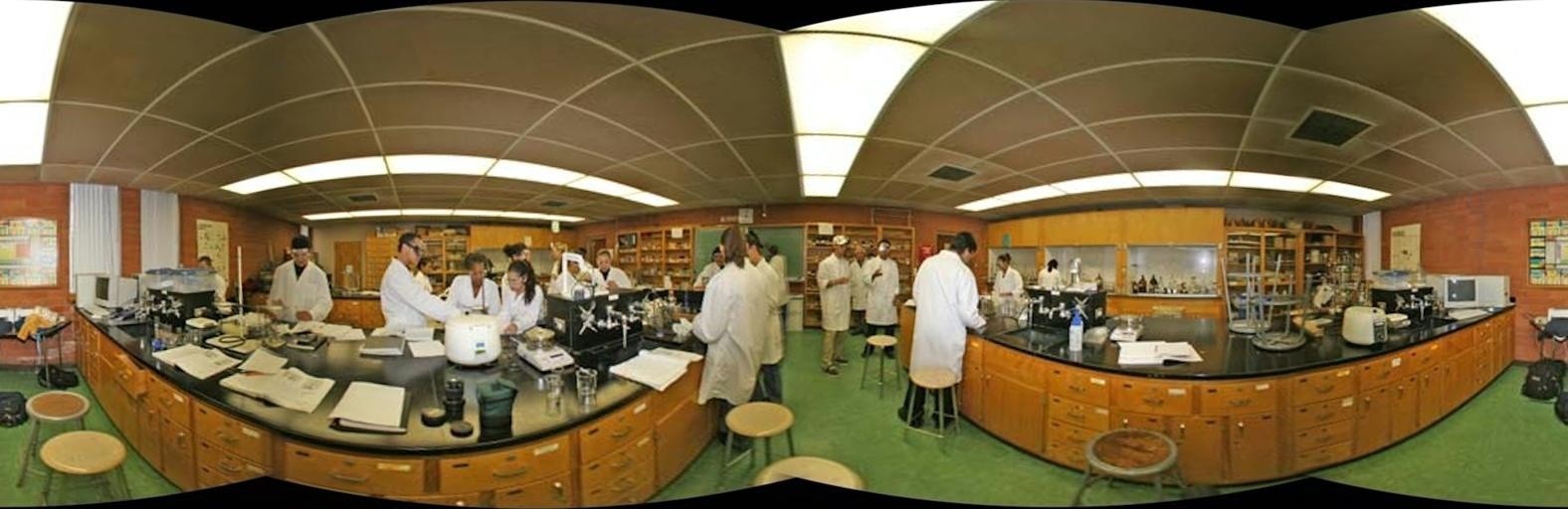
[833,235,849,246]
[746,230,764,248]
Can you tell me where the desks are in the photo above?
[1,313,71,388]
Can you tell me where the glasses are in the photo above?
[406,242,421,255]
[505,278,522,285]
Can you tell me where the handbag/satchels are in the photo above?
[37,365,78,389]
[0,392,27,427]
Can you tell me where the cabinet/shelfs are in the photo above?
[113,350,197,492]
[1280,358,1359,479]
[391,236,470,296]
[1440,324,1474,415]
[1474,319,1494,390]
[1299,229,1364,298]
[1494,306,1514,378]
[577,395,659,507]
[277,430,576,507]
[194,398,281,491]
[1120,297,1221,318]
[1354,339,1439,461]
[654,355,716,503]
[896,306,915,364]
[1044,362,1112,478]
[805,223,917,329]
[616,225,698,289]
[787,279,805,296]
[1110,373,1289,487]
[1221,226,1300,320]
[71,312,99,390]
[98,332,120,427]
[957,334,1048,460]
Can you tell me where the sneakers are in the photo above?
[826,367,839,375]
[835,357,849,364]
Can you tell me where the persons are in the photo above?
[691,225,768,449]
[991,252,1024,295]
[848,243,869,334]
[898,230,986,429]
[265,234,335,322]
[816,235,853,376]
[378,233,632,338]
[767,242,786,329]
[693,244,727,288]
[1037,258,1062,288]
[196,255,228,302]
[860,239,899,359]
[746,230,788,403]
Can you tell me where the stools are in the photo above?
[1220,252,1283,332]
[1073,428,1192,506]
[861,334,902,399]
[1249,272,1312,351]
[40,430,134,504]
[750,456,864,489]
[17,388,91,489]
[718,402,795,487]
[901,368,963,447]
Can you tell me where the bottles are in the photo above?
[1149,275,1157,293]
[1134,280,1138,292]
[1068,309,1084,352]
[1139,275,1146,293]
[1158,276,1163,289]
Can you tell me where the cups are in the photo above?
[545,400,561,417]
[577,398,596,416]
[544,375,564,399]
[576,367,598,399]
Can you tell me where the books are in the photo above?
[608,343,703,393]
[1119,340,1203,366]
[152,322,449,434]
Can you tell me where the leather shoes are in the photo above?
[898,408,924,427]
[935,418,953,429]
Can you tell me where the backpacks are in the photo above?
[1522,358,1564,399]
